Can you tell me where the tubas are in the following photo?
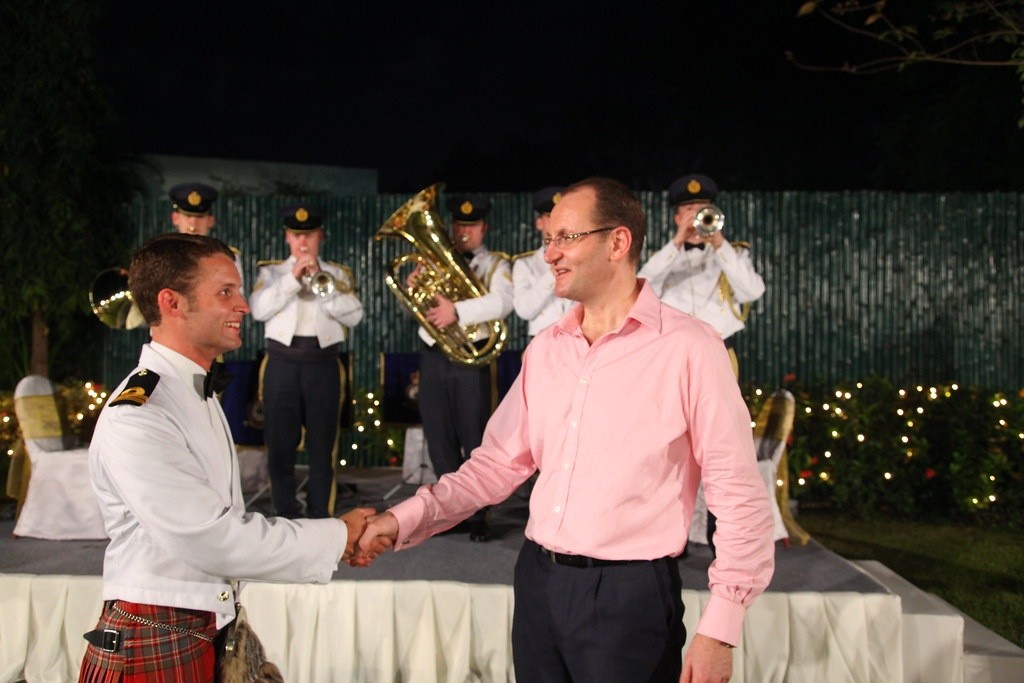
[374,182,509,366]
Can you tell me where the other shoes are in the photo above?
[470,521,488,542]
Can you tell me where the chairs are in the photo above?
[13,349,793,549]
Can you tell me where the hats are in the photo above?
[279,205,324,233]
[668,174,717,204]
[447,194,492,224]
[533,186,567,215]
[168,183,219,217]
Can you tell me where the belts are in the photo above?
[536,547,632,569]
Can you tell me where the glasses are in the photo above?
[543,226,618,248]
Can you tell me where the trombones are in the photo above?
[89,226,196,331]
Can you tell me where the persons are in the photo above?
[248,204,364,520]
[345,177,775,683]
[78,234,392,683]
[635,173,766,561]
[168,181,245,393]
[406,185,580,542]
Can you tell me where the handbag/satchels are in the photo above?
[222,604,284,683]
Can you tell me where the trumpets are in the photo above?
[694,205,726,235]
[300,245,336,297]
